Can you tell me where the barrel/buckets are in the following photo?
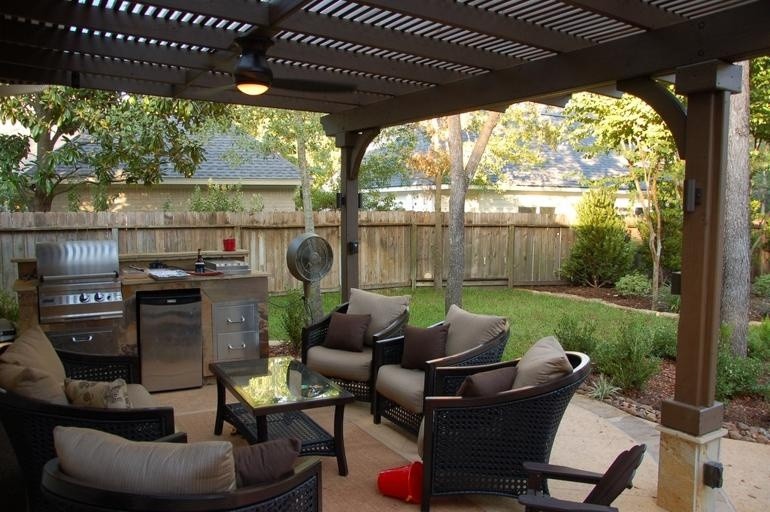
[378,461,424,502]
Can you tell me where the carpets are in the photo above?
[173,406,486,512]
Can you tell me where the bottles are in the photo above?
[195,249,205,272]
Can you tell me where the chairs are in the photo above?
[515,445,647,512]
[1,342,176,476]
[375,322,510,437]
[302,303,411,414]
[420,350,586,512]
[41,431,324,512]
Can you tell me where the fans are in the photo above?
[287,233,334,283]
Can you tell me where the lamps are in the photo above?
[235,36,277,96]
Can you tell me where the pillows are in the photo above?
[515,336,572,390]
[443,304,507,356]
[2,323,68,406]
[53,426,235,495]
[346,288,423,342]
[233,437,310,484]
[64,378,134,409]
[323,310,371,353]
[400,322,450,370]
[458,366,516,397]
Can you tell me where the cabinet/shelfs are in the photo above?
[9,249,271,392]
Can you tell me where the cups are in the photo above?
[223,238,236,251]
